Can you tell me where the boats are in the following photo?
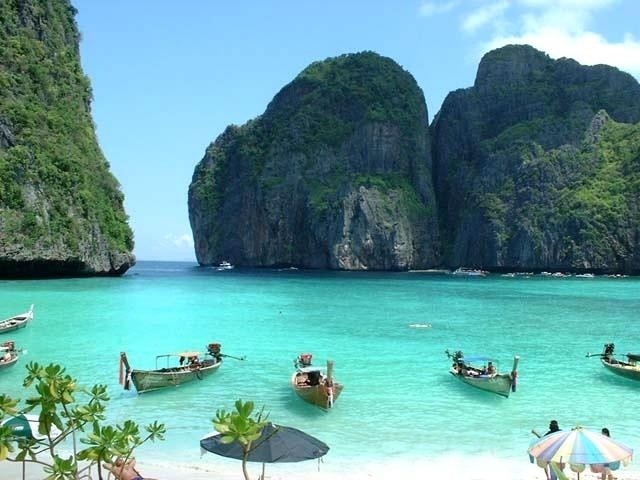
[120,343,223,395]
[600,343,640,381]
[449,350,519,398]
[292,354,344,409]
[0,303,34,333]
[0,340,18,366]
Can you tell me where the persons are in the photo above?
[601,427,611,479]
[184,355,202,370]
[103,458,156,480]
[543,419,564,480]
[487,361,496,374]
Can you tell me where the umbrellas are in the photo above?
[0,411,63,480]
[526,425,634,479]
[199,420,330,479]
